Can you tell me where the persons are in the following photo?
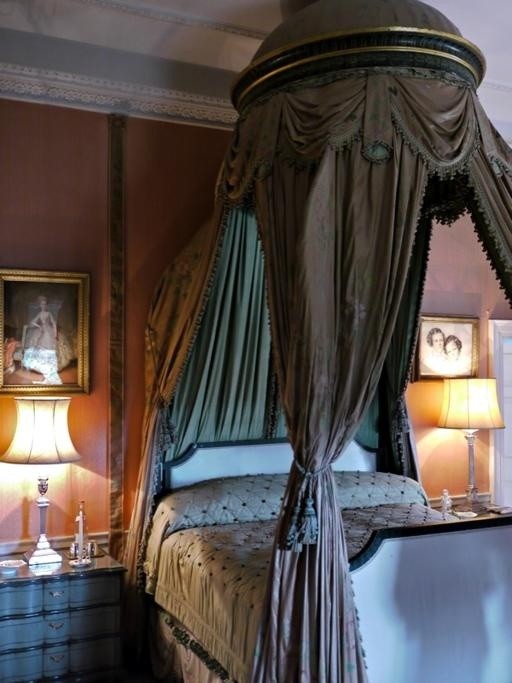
[441,333,463,361]
[425,326,445,352]
[30,293,61,375]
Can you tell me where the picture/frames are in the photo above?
[409,313,480,383]
[0,268,92,398]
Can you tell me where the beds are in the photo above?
[148,437,512,683]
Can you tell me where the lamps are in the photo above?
[436,377,505,514]
[0,396,82,566]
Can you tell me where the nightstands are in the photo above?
[0,546,128,683]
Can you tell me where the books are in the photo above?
[481,503,512,514]
